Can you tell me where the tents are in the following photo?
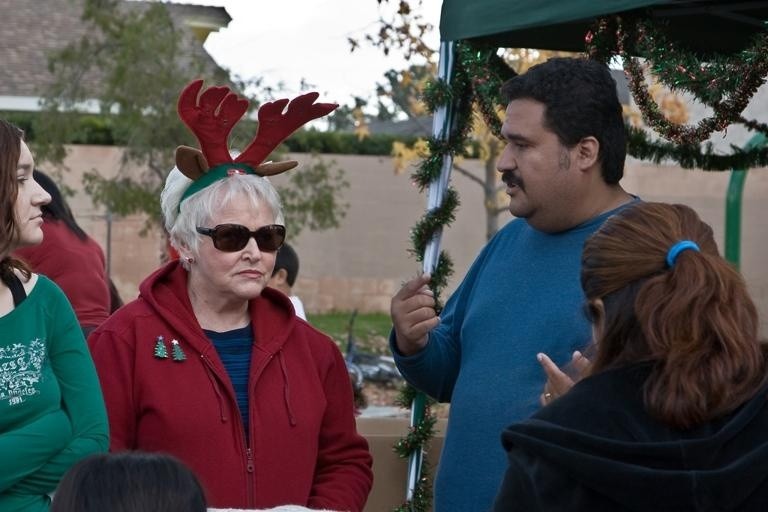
[404,0,768,511]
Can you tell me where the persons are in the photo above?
[0,122,110,511]
[87,147,373,511]
[492,203,768,511]
[266,241,306,320]
[9,169,111,336]
[49,452,208,512]
[392,56,647,512]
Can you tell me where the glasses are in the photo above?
[192,223,286,252]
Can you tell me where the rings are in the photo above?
[544,393,550,399]
